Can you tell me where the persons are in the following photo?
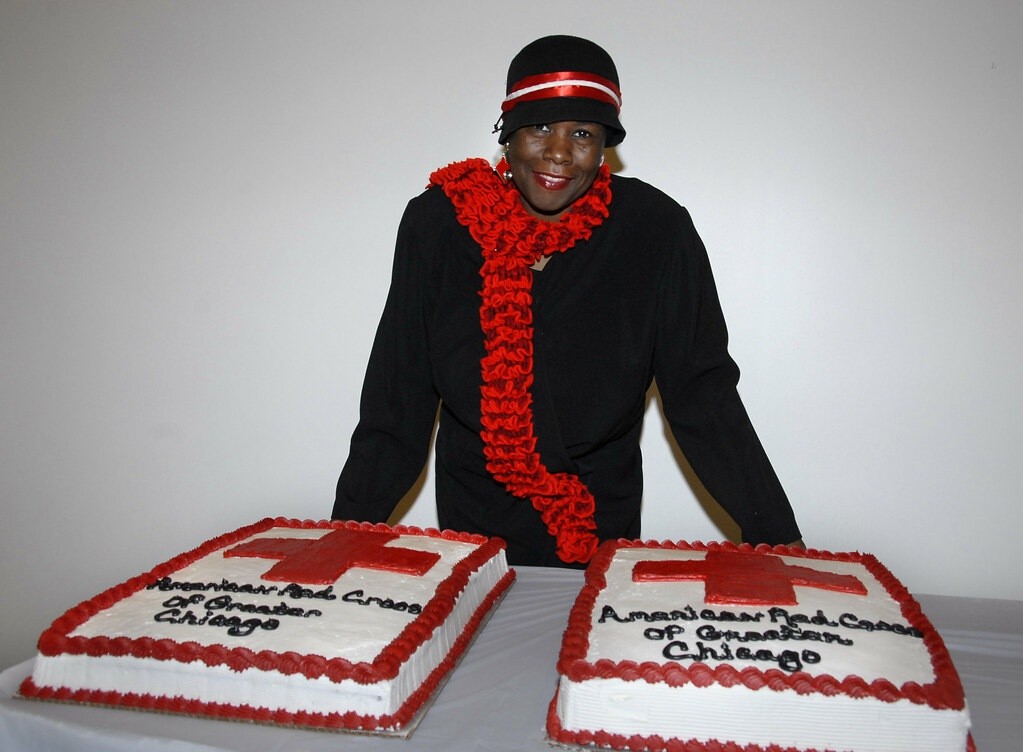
[331,35,804,570]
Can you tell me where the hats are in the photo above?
[492,35,626,148]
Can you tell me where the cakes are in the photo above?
[544,537,977,752]
[18,516,517,732]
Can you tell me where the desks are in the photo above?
[0,565,1023,752]
